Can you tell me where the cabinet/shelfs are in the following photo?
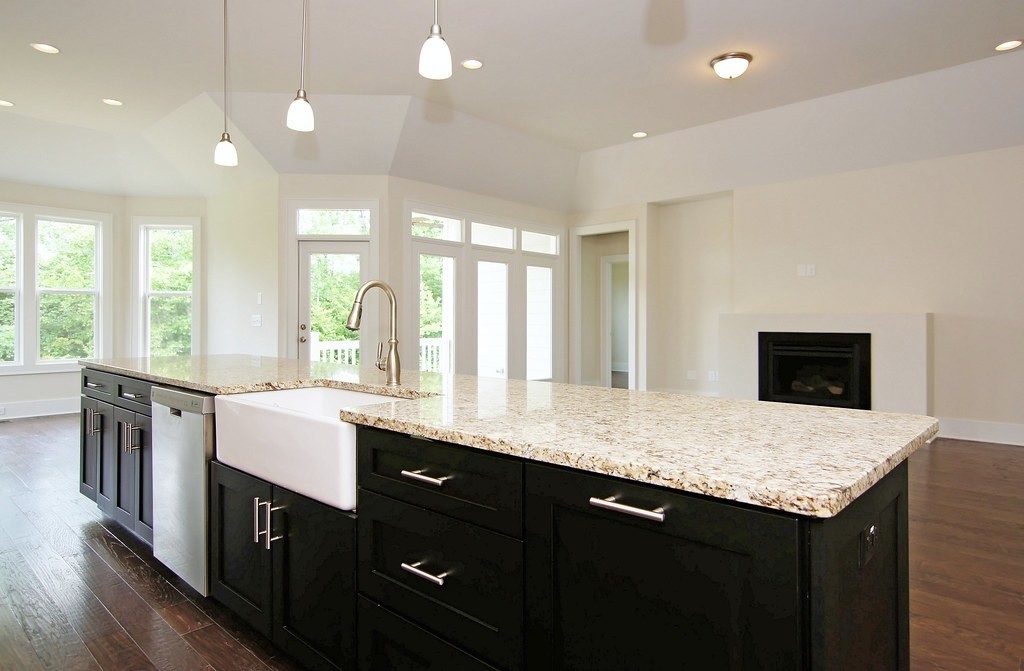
[210,460,358,671]
[355,426,525,671]
[80,368,158,548]
[524,457,910,671]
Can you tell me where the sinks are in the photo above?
[214,384,416,513]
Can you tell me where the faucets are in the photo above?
[345,278,401,387]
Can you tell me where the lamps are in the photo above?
[214,0,238,167]
[709,52,753,80]
[286,0,316,132]
[418,0,453,80]
